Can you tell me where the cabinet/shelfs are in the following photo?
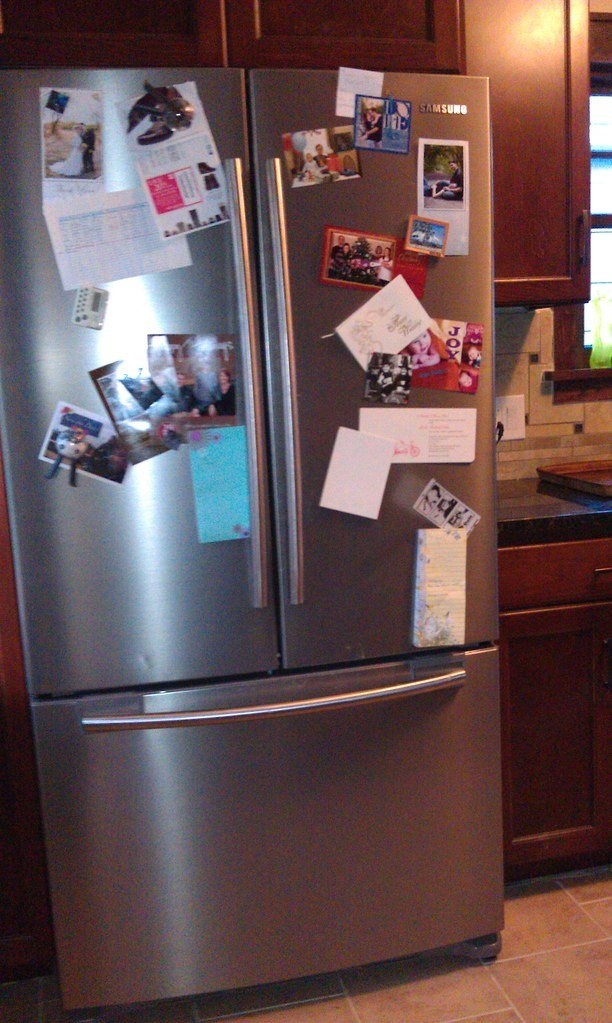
[497,535,612,893]
[0,0,593,309]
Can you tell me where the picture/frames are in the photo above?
[404,212,450,258]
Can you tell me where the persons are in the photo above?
[466,345,482,367]
[457,371,477,392]
[332,236,392,283]
[376,364,410,390]
[420,486,469,529]
[49,123,96,175]
[407,331,440,369]
[170,370,235,417]
[303,144,327,176]
[364,108,383,148]
[88,436,117,471]
[424,161,462,198]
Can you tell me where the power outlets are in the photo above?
[493,393,527,442]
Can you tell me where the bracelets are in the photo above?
[367,132,369,134]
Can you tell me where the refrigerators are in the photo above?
[0,70,503,1006]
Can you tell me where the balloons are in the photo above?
[283,132,307,152]
